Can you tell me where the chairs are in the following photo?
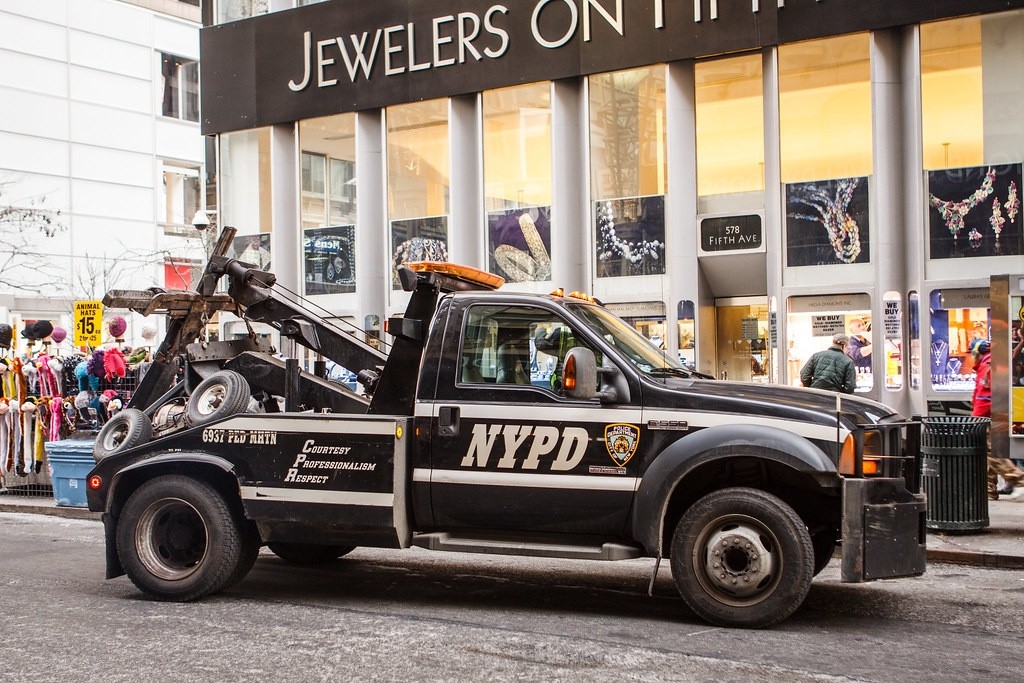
[495,341,530,384]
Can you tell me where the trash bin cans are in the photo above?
[918,414,992,531]
[45,437,102,508]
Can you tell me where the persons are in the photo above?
[1012,325,1024,360]
[801,334,856,394]
[970,339,1024,498]
[845,319,872,367]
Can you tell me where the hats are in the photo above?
[833,335,849,344]
[0,324,12,350]
[969,338,987,350]
[0,347,126,477]
[142,325,156,339]
[33,320,53,338]
[51,327,66,343]
[21,323,36,340]
[109,317,126,337]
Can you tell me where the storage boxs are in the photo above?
[43,438,98,508]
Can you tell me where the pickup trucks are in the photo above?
[83,226,929,631]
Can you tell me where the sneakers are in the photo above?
[1011,487,1024,500]
[988,492,999,500]
